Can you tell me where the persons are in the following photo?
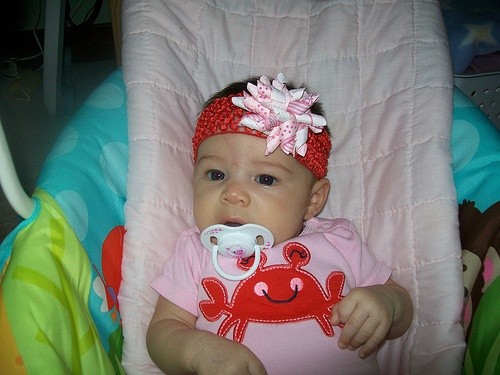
[146,76,414,375]
